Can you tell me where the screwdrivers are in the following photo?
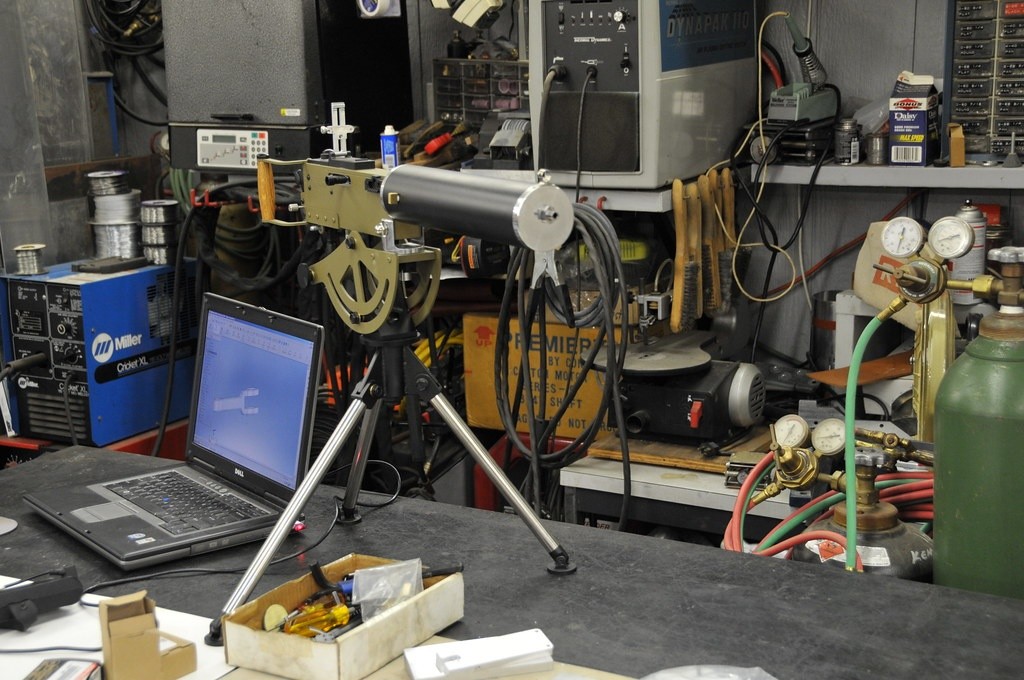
[285,602,362,639]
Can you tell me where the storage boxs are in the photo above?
[403,628,554,680]
[220,553,463,680]
[98,590,197,680]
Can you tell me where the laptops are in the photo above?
[23,292,324,570]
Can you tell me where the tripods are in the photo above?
[203,229,578,646]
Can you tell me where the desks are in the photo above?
[560,457,934,549]
[0,443,1024,680]
